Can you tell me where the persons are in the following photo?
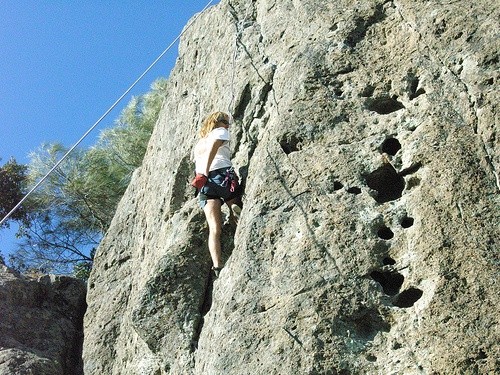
[190,112,243,277]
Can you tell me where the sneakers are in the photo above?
[211,266,223,277]
[230,203,242,219]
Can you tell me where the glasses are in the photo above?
[219,119,230,125]
